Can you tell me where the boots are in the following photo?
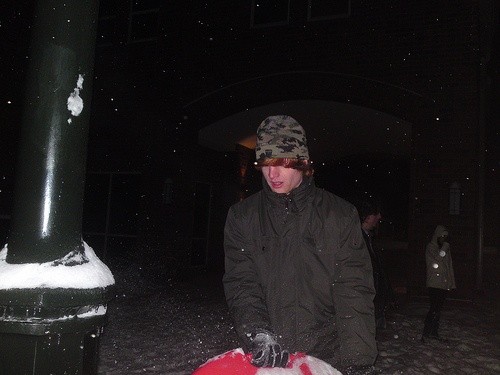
[421,313,449,343]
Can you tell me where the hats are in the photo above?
[254,114,310,171]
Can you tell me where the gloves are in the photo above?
[242,331,289,368]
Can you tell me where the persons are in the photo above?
[421,226,456,344]
[358,204,391,342]
[223,115,382,375]
